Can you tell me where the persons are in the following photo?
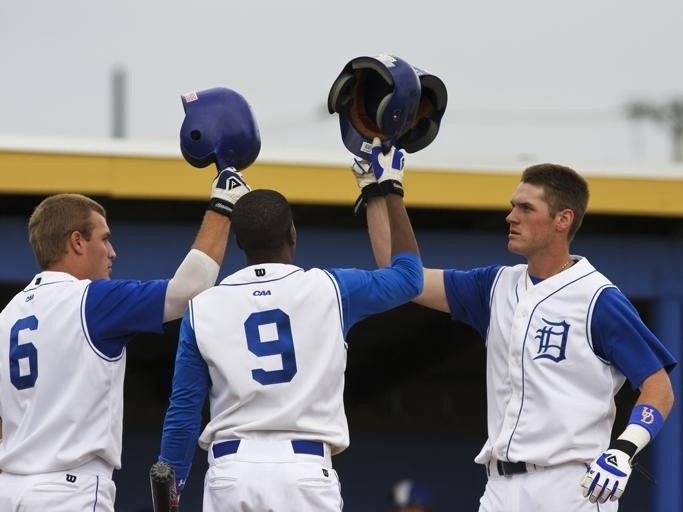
[0,166,251,512]
[349,154,677,512]
[151,137,425,512]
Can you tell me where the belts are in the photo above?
[497,459,527,475]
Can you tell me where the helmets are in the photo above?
[180,87,261,172]
[328,53,448,162]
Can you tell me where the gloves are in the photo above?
[580,439,639,503]
[207,167,252,218]
[351,137,407,216]
